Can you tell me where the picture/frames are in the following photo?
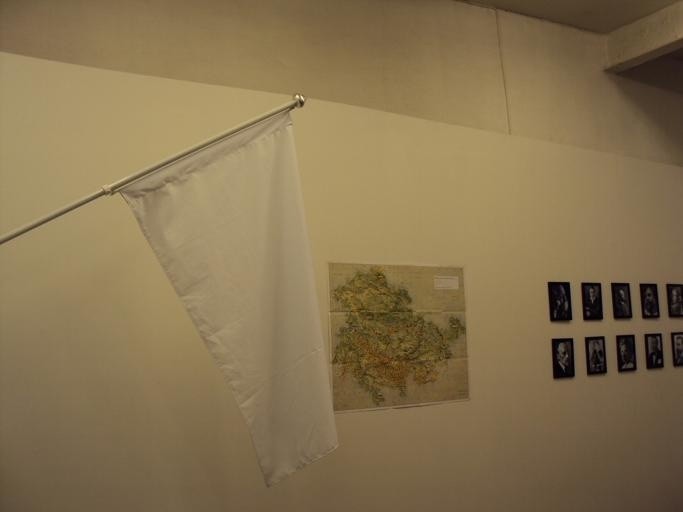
[547,282,683,378]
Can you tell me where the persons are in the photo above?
[545,280,683,381]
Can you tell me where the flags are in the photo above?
[118,107,344,485]
